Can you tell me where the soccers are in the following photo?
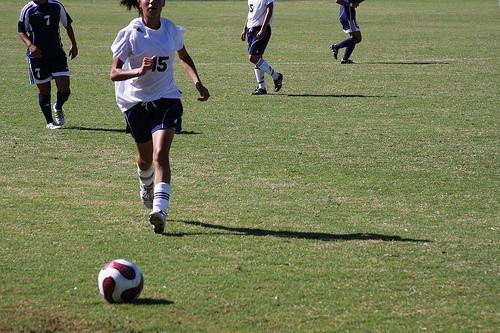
[98,259,144,304]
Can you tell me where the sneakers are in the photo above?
[330,44,337,60]
[53,103,64,125]
[46,121,61,130]
[274,73,283,92]
[140,180,154,209]
[340,58,356,64]
[252,88,267,95]
[148,210,166,234]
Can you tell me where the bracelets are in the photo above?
[27,44,32,47]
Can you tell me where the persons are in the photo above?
[329,0,364,63]
[17,0,78,129]
[241,0,283,95]
[110,0,210,233]
